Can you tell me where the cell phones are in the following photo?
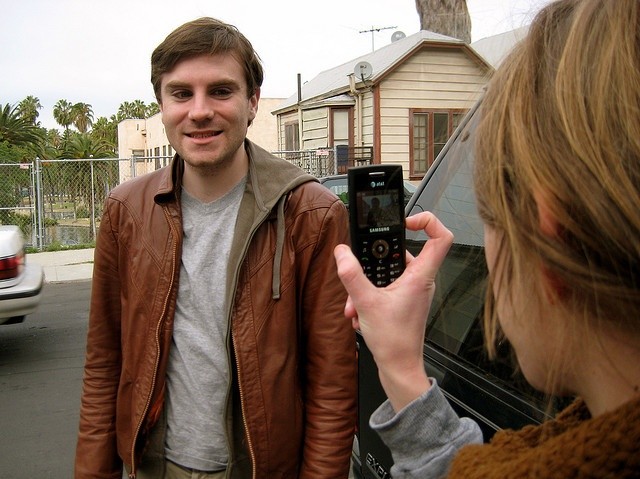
[348,164,406,288]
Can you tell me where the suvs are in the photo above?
[349,85,574,478]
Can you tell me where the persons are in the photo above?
[365,197,389,226]
[73,17,359,479]
[334,0,639,479]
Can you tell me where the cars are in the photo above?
[315,170,418,207]
[0,224,45,325]
